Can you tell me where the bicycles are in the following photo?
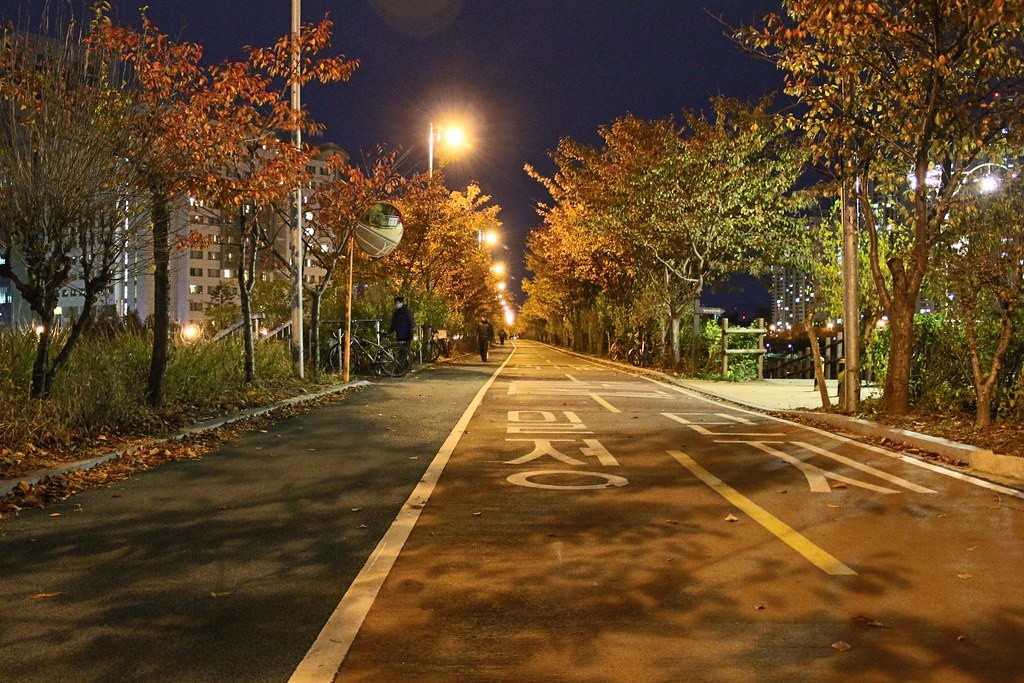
[609,339,621,362]
[329,320,412,379]
[419,325,451,363]
[628,332,653,367]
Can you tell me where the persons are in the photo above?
[498,330,507,345]
[388,297,413,374]
[476,317,493,362]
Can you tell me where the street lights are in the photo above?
[424,122,466,292]
